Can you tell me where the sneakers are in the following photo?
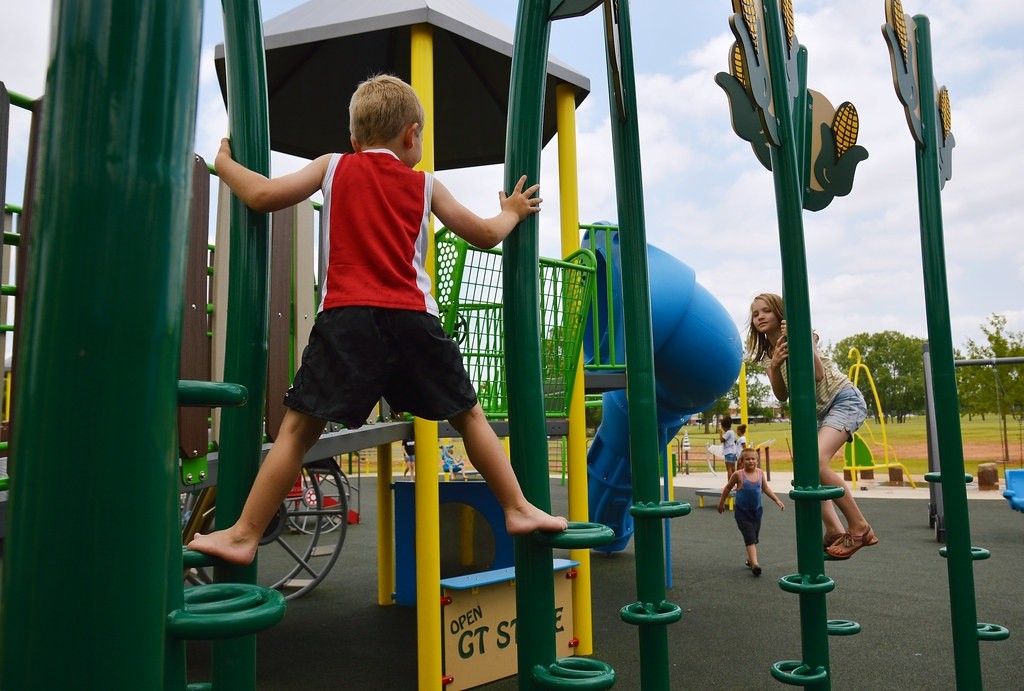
[751,565,762,574]
[745,559,752,568]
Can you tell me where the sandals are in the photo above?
[827,525,879,559]
[823,532,846,551]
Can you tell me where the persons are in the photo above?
[734,423,747,469]
[742,294,878,558]
[719,416,737,490]
[718,448,785,577]
[402,439,415,481]
[187,75,568,565]
[445,449,468,480]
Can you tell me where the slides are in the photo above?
[575,219,742,554]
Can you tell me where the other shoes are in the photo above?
[720,485,736,493]
